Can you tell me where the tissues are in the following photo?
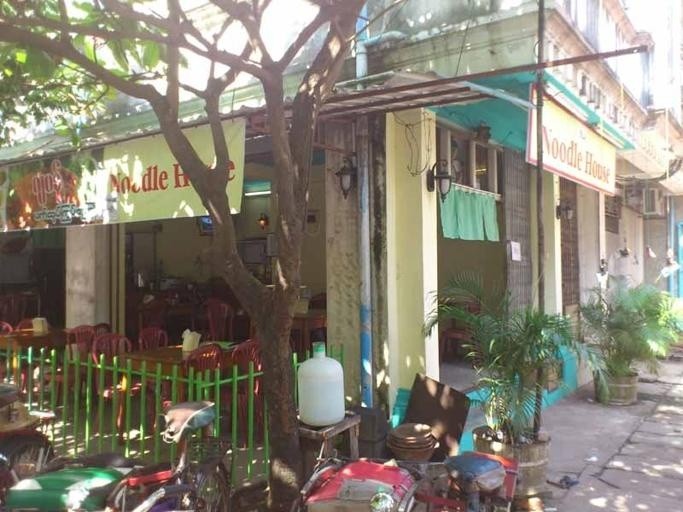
[181,328,202,352]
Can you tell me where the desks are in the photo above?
[129,298,200,348]
[236,307,331,375]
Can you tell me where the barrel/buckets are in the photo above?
[297,341,347,429]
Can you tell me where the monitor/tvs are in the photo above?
[197,216,214,236]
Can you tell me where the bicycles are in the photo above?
[137,399,231,512]
[0,426,54,511]
[424,449,520,511]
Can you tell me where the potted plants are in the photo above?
[422,271,584,500]
[573,282,683,408]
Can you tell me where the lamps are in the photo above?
[333,156,358,200]
[556,196,574,223]
[427,158,453,204]
[255,213,268,229]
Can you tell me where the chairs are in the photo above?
[205,299,247,366]
[306,294,336,369]
[441,300,481,367]
[0,319,264,446]
